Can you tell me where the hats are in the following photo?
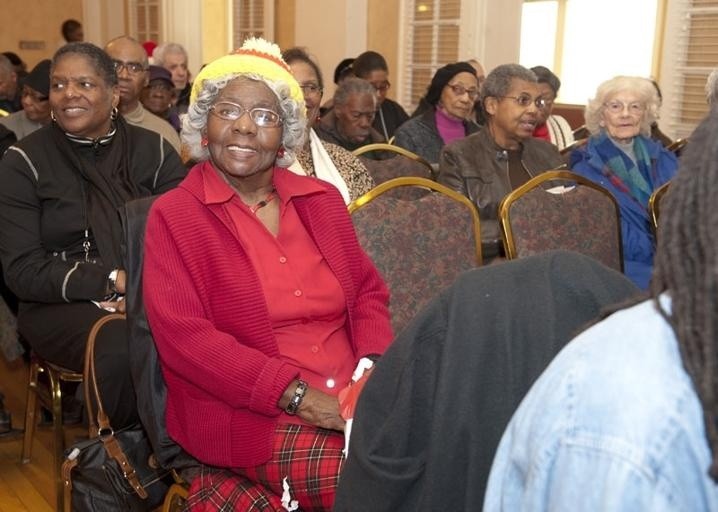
[148,65,176,87]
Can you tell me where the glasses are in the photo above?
[112,61,144,73]
[503,95,545,109]
[447,85,477,96]
[605,102,644,112]
[208,102,283,128]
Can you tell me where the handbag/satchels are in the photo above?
[61,421,175,512]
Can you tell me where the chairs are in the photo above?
[17,349,90,511]
[344,177,483,344]
[334,251,650,511]
[354,143,437,185]
[498,168,626,280]
[649,181,667,244]
[116,193,199,511]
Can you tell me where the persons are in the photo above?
[479,70,718,512]
[2,14,684,512]
